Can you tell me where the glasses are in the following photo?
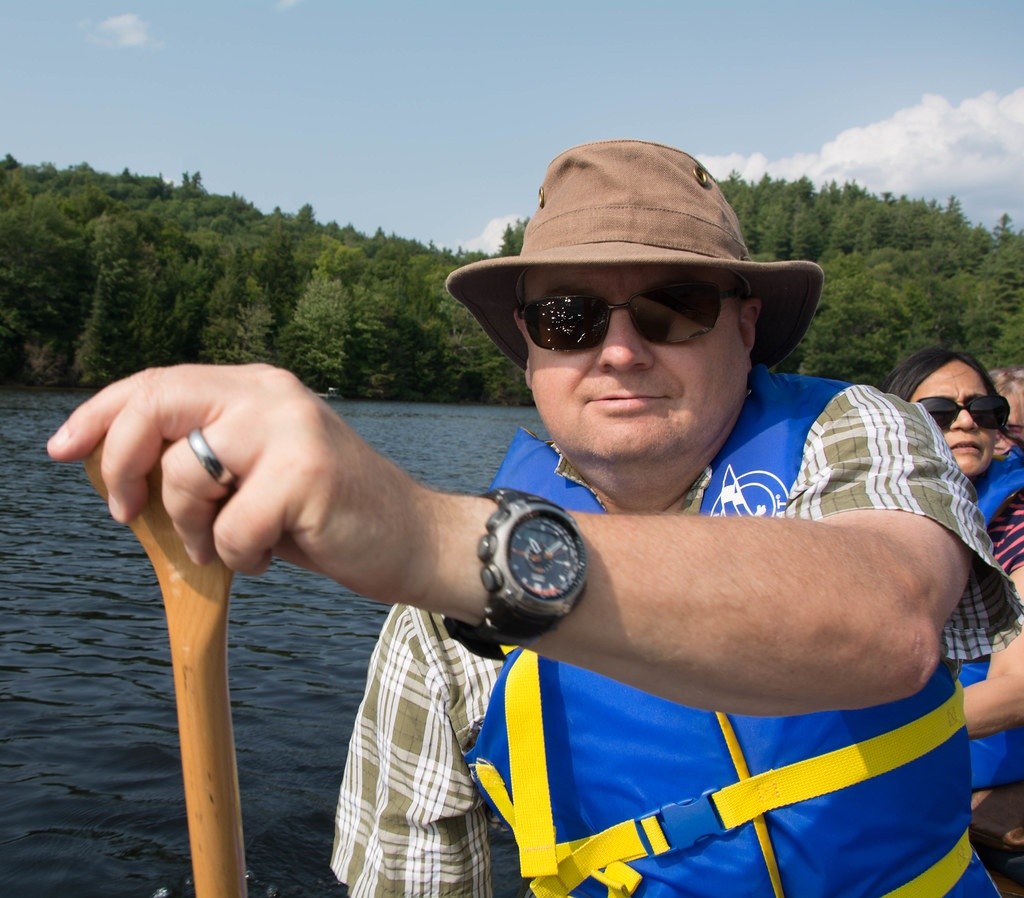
[913,396,1010,431]
[518,280,742,352]
[997,424,1024,444]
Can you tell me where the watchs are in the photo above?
[443,486,593,662]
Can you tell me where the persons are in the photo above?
[982,368,1022,448]
[42,136,1023,898]
[880,345,1022,788]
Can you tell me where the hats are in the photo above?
[445,141,825,373]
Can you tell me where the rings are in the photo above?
[183,425,239,492]
